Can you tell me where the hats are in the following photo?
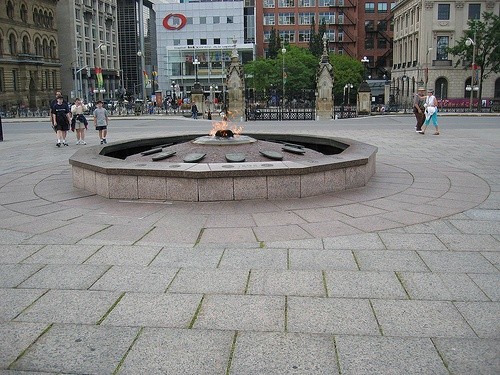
[418,86,426,90]
[95,100,103,104]
[56,95,63,99]
[428,88,434,95]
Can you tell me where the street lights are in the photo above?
[75,64,91,99]
[361,56,370,79]
[425,47,434,98]
[193,59,201,83]
[342,85,348,107]
[209,83,218,105]
[171,80,176,106]
[464,32,477,112]
[347,83,353,106]
[282,47,287,108]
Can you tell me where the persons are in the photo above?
[70,98,87,145]
[93,101,109,144]
[51,95,72,147]
[413,87,425,132]
[163,96,188,114]
[50,91,68,138]
[412,89,439,135]
[192,101,198,120]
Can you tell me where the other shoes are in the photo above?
[82,141,86,145]
[76,140,80,145]
[56,142,61,147]
[432,132,439,135]
[416,130,422,132]
[419,131,424,134]
[62,140,69,146]
[100,140,103,144]
[104,138,107,144]
